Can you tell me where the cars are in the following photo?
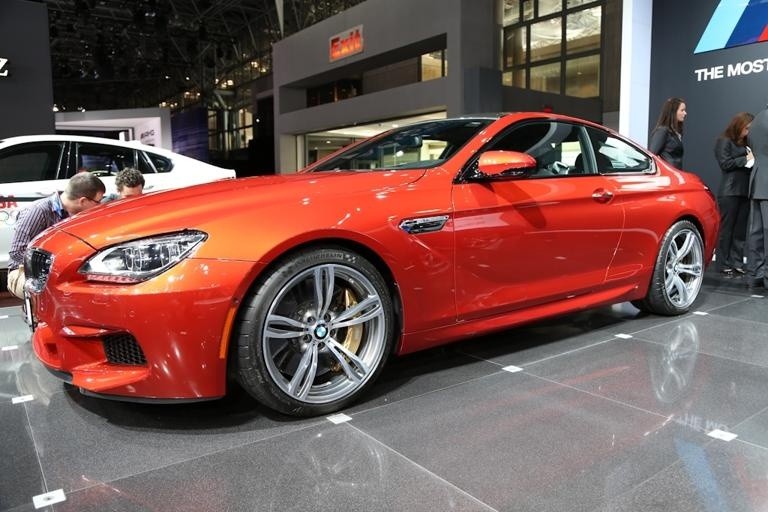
[13,105,725,431]
[0,126,245,291]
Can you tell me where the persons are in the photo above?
[747,107,768,302]
[713,110,754,276]
[649,96,688,171]
[116,167,145,199]
[6,170,107,301]
